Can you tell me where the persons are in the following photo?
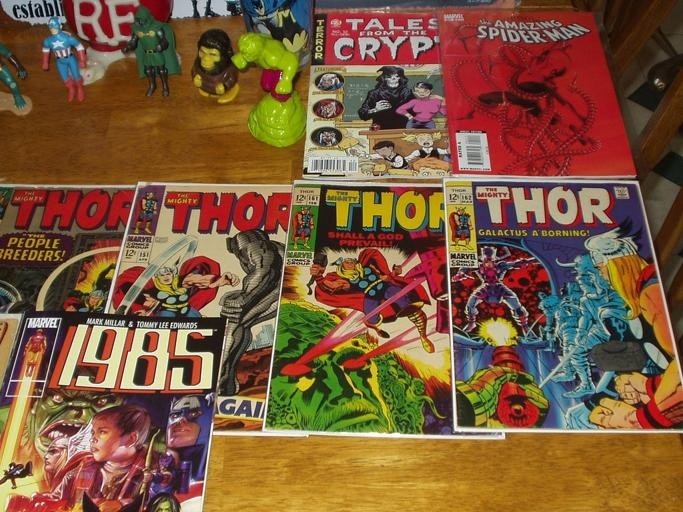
[42,17,86,102]
[121,5,182,98]
[1,46,28,110]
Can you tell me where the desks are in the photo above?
[0,2,683,512]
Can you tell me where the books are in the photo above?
[2,183,287,511]
[262,7,683,439]
